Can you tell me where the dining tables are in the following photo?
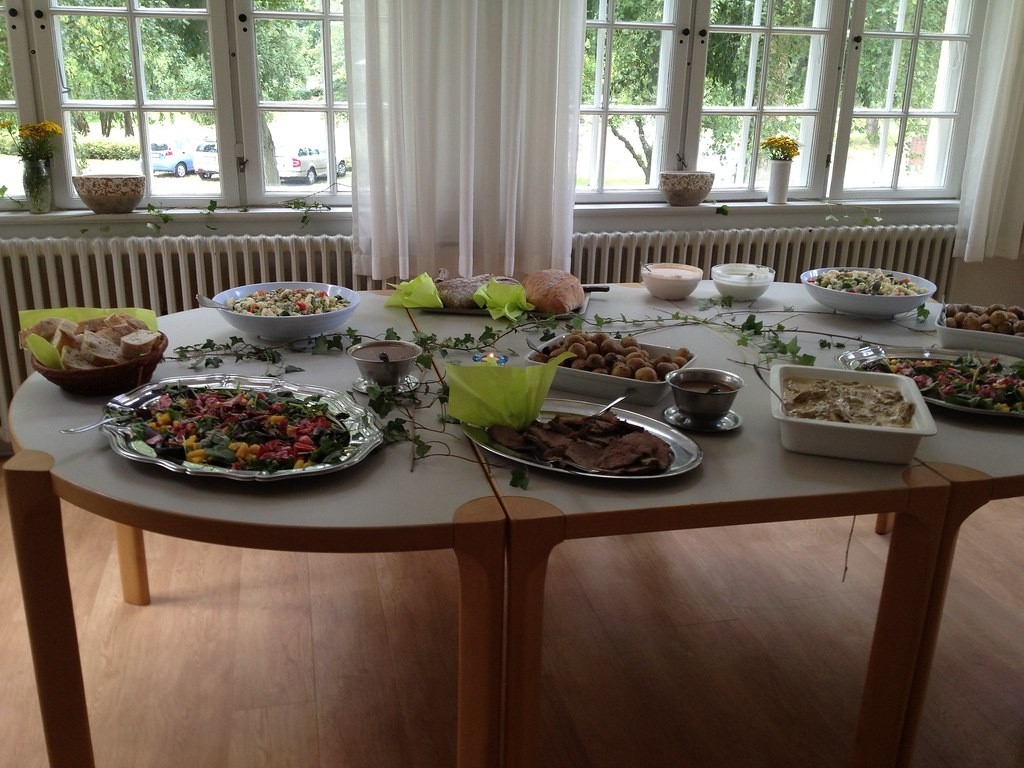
[2,280,1023,767]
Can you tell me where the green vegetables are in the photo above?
[855,356,1024,410]
[107,381,360,470]
[813,268,909,295]
[239,288,350,316]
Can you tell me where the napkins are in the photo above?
[383,270,443,309]
[473,273,537,324]
[442,350,578,428]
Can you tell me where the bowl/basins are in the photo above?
[658,170,716,206]
[640,262,703,301]
[800,267,937,318]
[212,281,360,341]
[934,303,1024,358]
[710,263,776,301]
[770,364,937,464]
[71,174,145,214]
[665,367,746,427]
[524,333,697,407]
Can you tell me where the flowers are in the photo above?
[762,134,800,161]
[1,121,63,198]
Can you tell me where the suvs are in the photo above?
[192,135,220,179]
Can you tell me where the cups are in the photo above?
[347,340,422,388]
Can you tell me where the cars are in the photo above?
[274,147,346,184]
[140,142,195,177]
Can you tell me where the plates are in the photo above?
[98,374,385,481]
[833,348,1024,419]
[352,375,420,394]
[460,398,704,480]
[662,405,742,433]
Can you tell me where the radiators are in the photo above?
[0,232,423,461]
[568,223,965,308]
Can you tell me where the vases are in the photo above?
[23,160,53,214]
[72,175,144,213]
[659,172,715,208]
[768,159,791,204]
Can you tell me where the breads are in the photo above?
[19,312,161,369]
[436,270,584,312]
